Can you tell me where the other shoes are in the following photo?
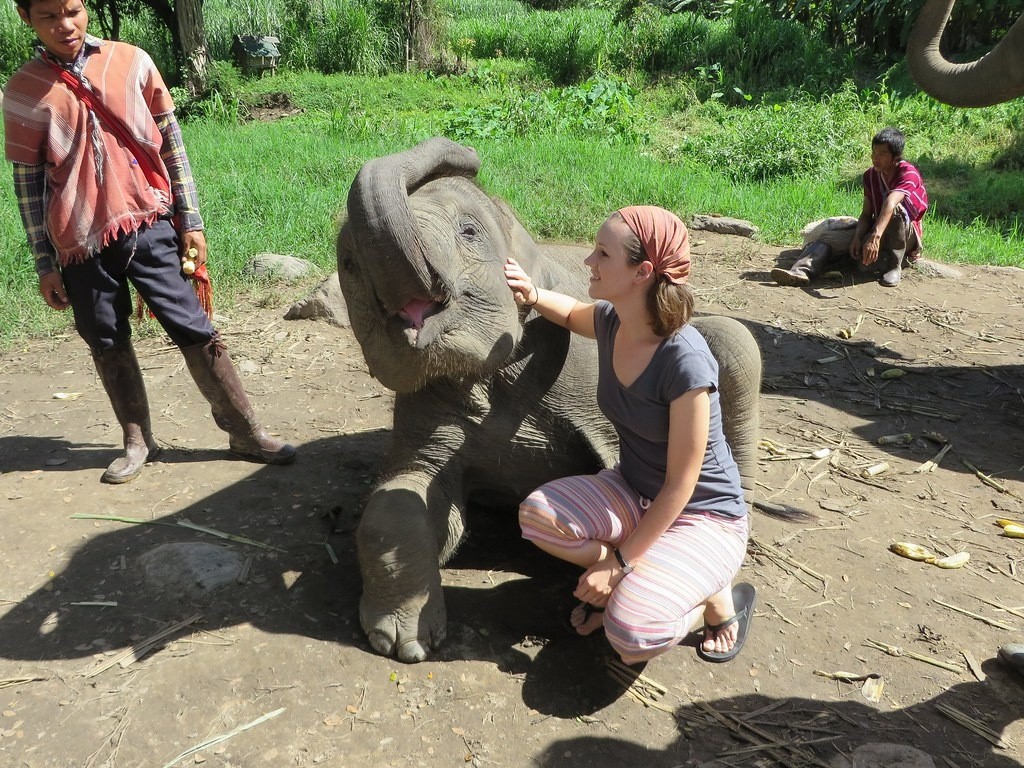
[998,643,1024,684]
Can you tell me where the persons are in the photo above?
[2,0,296,483]
[771,128,929,287]
[504,206,756,663]
[229,34,244,66]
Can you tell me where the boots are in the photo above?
[91,337,158,484]
[770,239,832,287]
[181,330,296,464]
[881,214,906,287]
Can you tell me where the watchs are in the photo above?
[614,548,635,573]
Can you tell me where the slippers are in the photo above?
[699,583,757,662]
[563,602,605,640]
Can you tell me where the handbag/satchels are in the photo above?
[137,209,214,323]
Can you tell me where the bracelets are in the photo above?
[524,284,538,307]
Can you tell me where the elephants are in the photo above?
[906,0,1024,109]
[337,135,762,665]
